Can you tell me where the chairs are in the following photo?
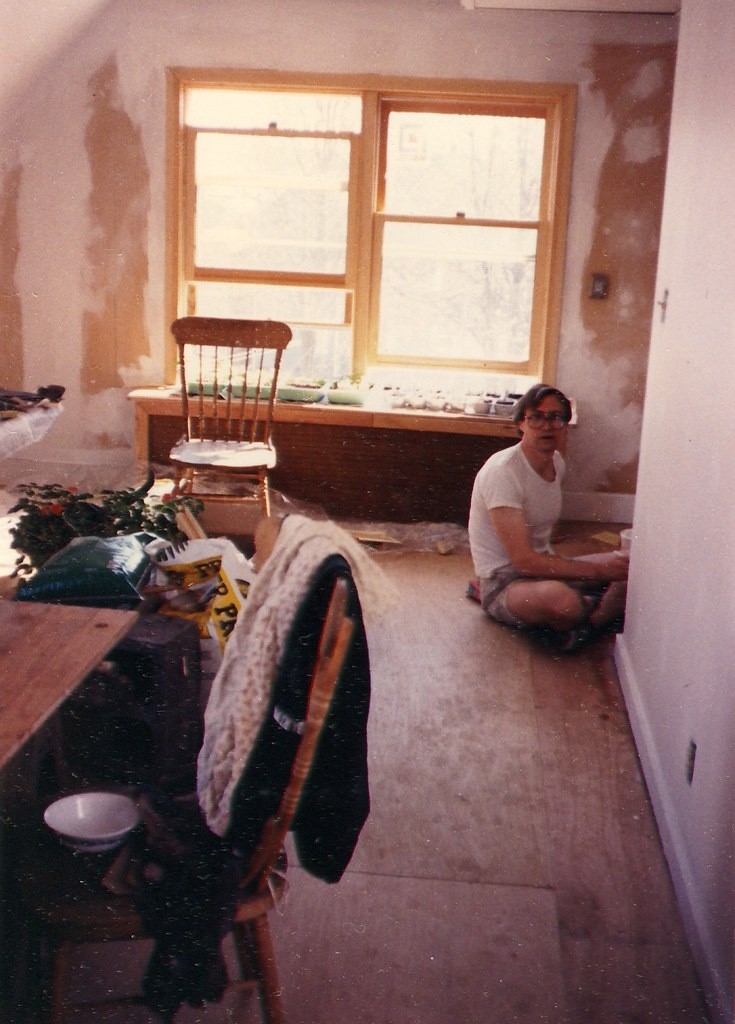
[171,317,291,519]
[13,514,354,1024]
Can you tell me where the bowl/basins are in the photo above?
[44,792,140,851]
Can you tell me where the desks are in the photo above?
[127,383,578,527]
[0,600,140,829]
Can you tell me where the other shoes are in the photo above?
[536,621,576,652]
[582,594,602,611]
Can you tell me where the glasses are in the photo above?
[522,412,569,431]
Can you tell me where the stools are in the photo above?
[99,612,203,779]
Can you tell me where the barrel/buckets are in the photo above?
[619,528,632,551]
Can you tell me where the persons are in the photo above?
[467,383,631,651]
